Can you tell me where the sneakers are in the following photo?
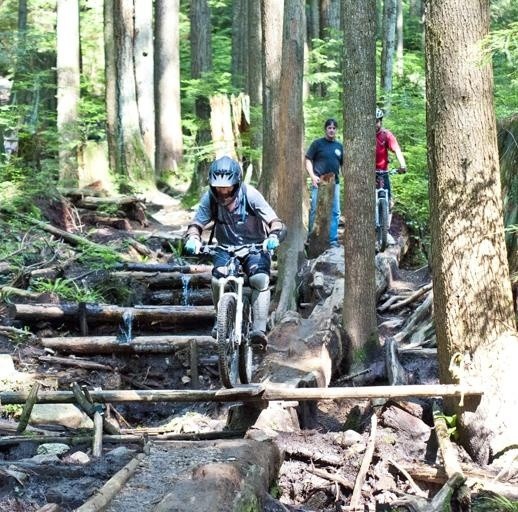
[386,233,396,246]
[251,330,269,355]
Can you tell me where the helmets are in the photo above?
[375,107,384,120]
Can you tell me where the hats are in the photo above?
[207,155,243,208]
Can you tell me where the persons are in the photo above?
[375,106,409,247]
[185,156,287,355]
[302,118,346,248]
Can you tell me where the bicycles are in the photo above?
[198,243,265,389]
[374,168,407,252]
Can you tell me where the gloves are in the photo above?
[262,236,281,254]
[183,233,202,255]
